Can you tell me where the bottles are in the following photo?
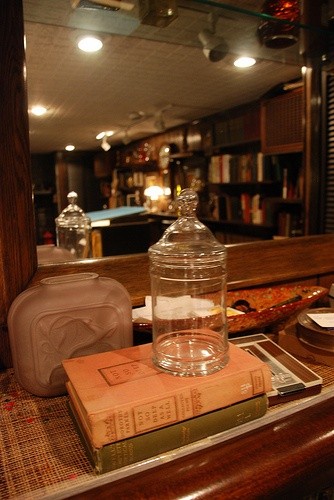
[55,192,92,261]
[148,189,227,377]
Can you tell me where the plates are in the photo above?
[131,285,329,335]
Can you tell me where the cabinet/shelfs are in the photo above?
[100,84,309,237]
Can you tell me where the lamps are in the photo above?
[195,14,231,65]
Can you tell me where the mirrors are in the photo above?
[0,0,334,307]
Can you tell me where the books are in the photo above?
[222,334,322,407]
[61,327,273,474]
[82,206,148,221]
[208,192,279,225]
[206,152,285,183]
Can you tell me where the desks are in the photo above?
[0,284,332,499]
